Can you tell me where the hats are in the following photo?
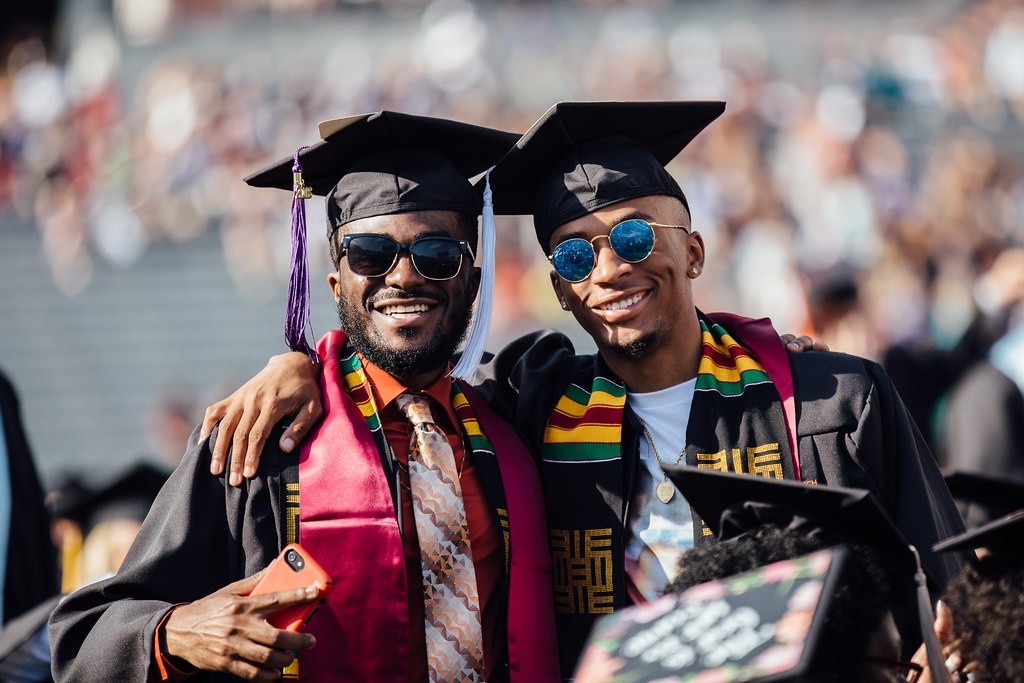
[659,461,950,683]
[448,101,727,382]
[242,111,523,364]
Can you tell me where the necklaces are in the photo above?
[636,416,686,504]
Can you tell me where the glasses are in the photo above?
[336,233,475,281]
[548,219,691,283]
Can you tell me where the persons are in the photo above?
[199,100,979,683]
[47,108,830,683]
[0,0,1024,683]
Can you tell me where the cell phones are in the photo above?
[248,543,333,634]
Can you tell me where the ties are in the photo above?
[396,393,484,682]
